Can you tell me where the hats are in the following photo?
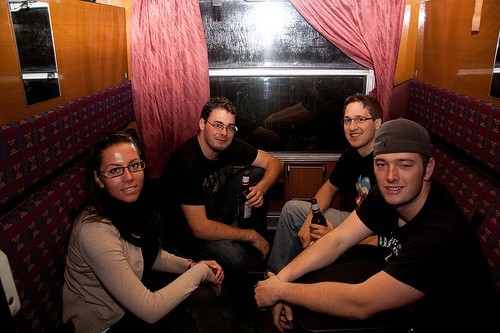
[373,117,433,157]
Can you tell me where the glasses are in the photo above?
[340,116,375,126]
[202,117,238,134]
[95,160,146,179]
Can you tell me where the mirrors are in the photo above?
[488,30,500,100]
[7,0,62,107]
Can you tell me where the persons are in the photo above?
[232,80,354,151]
[254,120,500,333]
[62,134,225,332]
[267,91,384,273]
[158,97,283,294]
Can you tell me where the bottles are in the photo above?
[310,198,326,227]
[237,172,253,220]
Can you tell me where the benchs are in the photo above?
[406,79,500,298]
[0,78,135,332]
